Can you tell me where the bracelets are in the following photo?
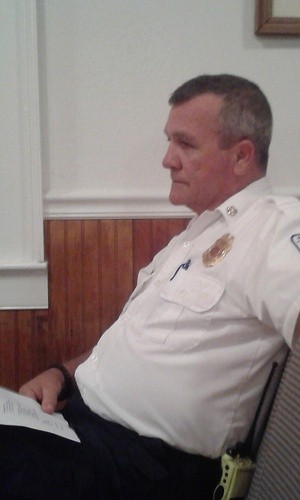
[46,363,75,401]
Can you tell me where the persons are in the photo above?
[1,74,300,500]
